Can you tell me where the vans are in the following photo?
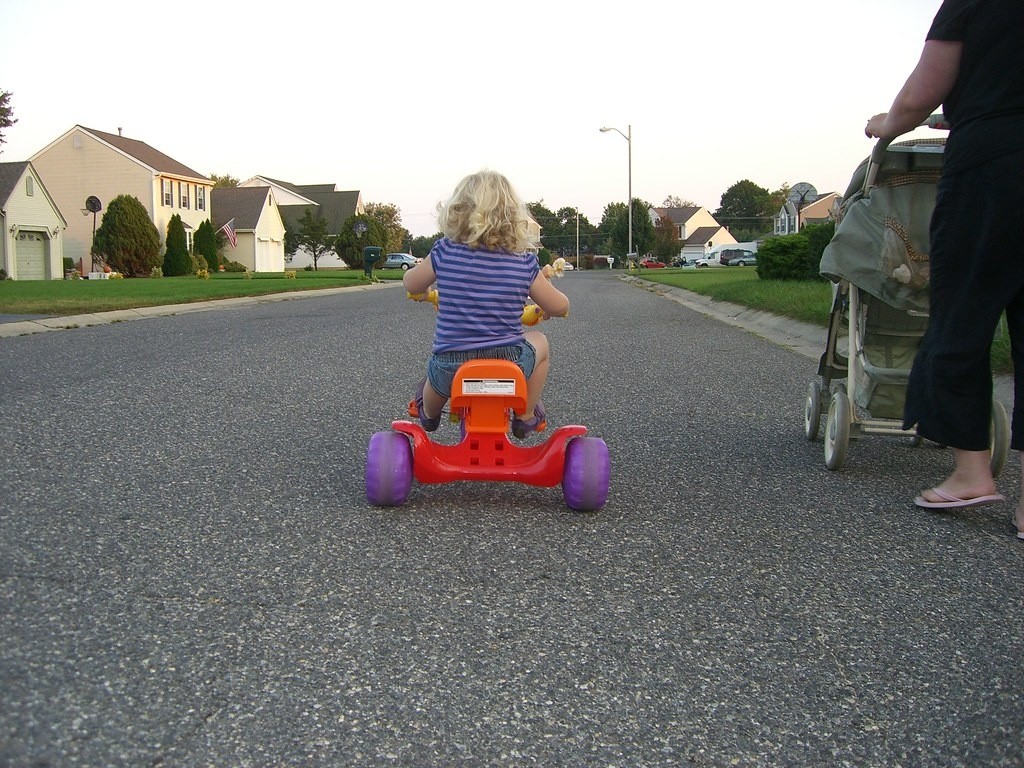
[695,242,757,268]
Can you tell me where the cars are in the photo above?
[564,261,574,271]
[728,253,757,267]
[684,259,698,268]
[383,253,422,271]
[639,259,665,269]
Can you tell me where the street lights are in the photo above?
[598,125,633,271]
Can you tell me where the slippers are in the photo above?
[913,488,1006,508]
[1012,506,1024,539]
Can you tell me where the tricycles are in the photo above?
[361,289,611,513]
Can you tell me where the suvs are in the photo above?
[719,249,754,266]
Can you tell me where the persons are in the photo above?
[403,170,570,438]
[865,0,1024,539]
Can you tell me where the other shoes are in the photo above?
[416,376,442,431]
[513,399,546,439]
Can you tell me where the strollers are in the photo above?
[806,119,1013,479]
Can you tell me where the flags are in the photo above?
[223,220,238,248]
